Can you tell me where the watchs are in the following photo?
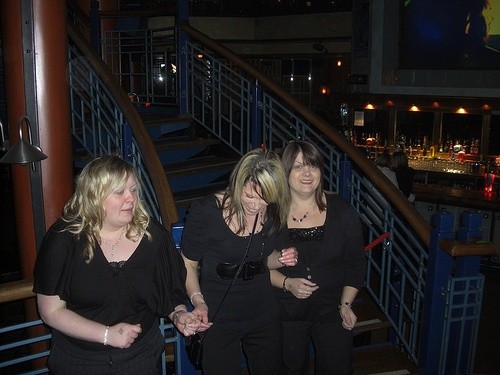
[172,310,187,325]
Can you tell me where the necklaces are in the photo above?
[288,203,315,223]
[246,212,263,235]
[105,229,125,256]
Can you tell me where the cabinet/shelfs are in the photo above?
[414,182,500,272]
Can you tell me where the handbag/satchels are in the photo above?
[408,193,415,203]
[185,331,203,370]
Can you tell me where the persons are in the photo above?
[266,140,369,375]
[180,149,298,375]
[32,155,200,375]
[352,149,417,226]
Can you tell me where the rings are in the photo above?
[306,291,309,295]
[194,333,196,335]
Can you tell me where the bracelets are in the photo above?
[338,302,352,312]
[190,293,203,306]
[277,252,285,266]
[104,326,109,345]
[283,275,288,293]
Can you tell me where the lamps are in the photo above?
[0,116,48,171]
[313,43,325,51]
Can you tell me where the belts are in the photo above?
[216,259,267,281]
